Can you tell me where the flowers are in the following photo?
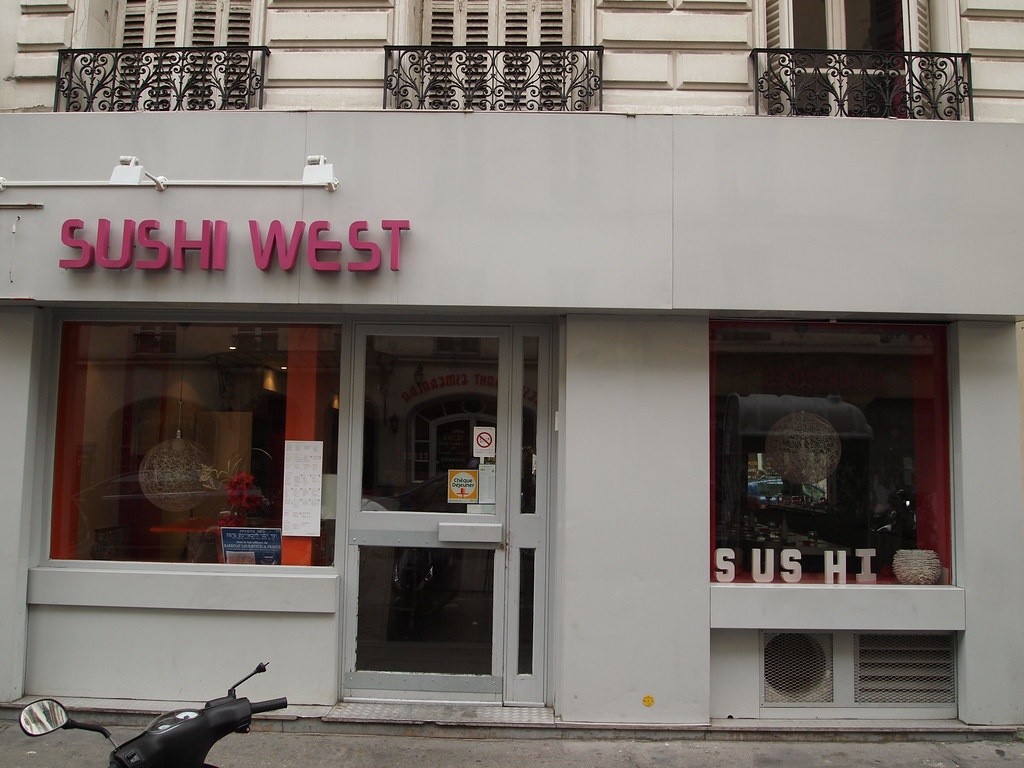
[204,444,276,539]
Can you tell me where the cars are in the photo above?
[71,468,272,560]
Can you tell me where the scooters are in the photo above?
[387,549,453,640]
[19,662,288,768]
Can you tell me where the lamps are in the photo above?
[109,155,170,191]
[757,322,844,485]
[134,324,213,515]
[302,157,340,190]
